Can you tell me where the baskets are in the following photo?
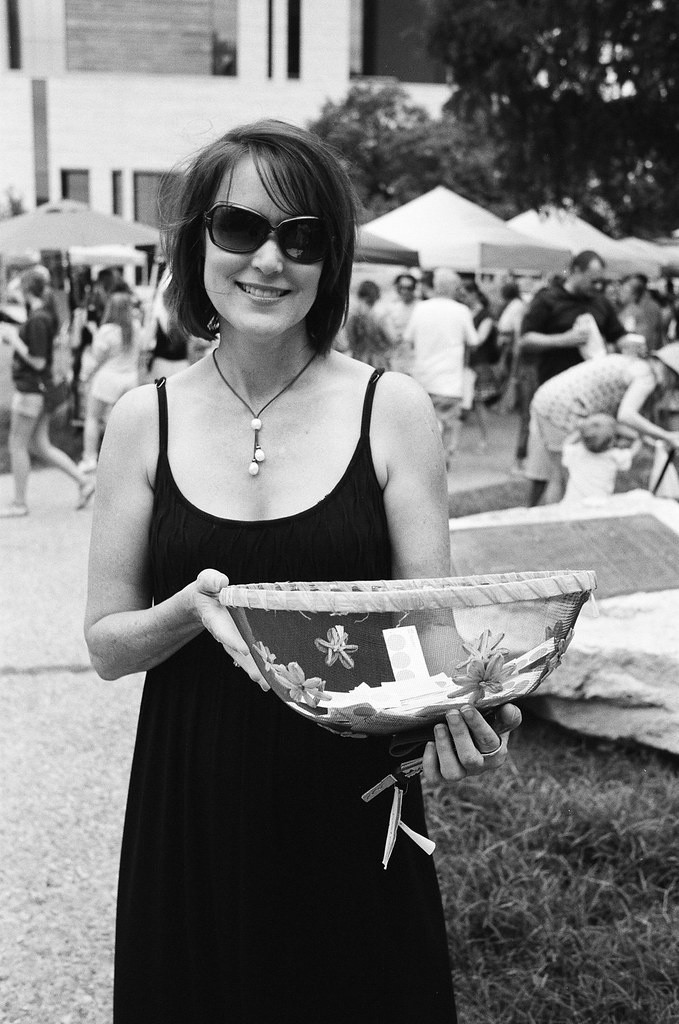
[220,570,600,733]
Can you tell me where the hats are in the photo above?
[651,340,679,374]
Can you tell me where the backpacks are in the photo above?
[346,307,378,357]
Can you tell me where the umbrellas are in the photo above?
[0,199,163,251]
[351,227,420,267]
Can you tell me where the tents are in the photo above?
[617,236,679,273]
[506,209,662,278]
[363,186,571,273]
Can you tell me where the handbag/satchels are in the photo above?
[42,382,70,412]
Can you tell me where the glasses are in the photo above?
[198,202,334,263]
[398,285,413,291]
[583,268,598,285]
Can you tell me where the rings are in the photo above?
[481,733,503,757]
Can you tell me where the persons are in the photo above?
[84,119,522,1024]
[0,262,217,517]
[336,250,679,470]
[561,415,644,503]
[525,343,679,507]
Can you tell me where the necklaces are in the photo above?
[213,348,319,475]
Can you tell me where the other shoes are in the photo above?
[79,457,98,472]
[75,482,96,511]
[0,503,29,518]
[513,464,526,476]
